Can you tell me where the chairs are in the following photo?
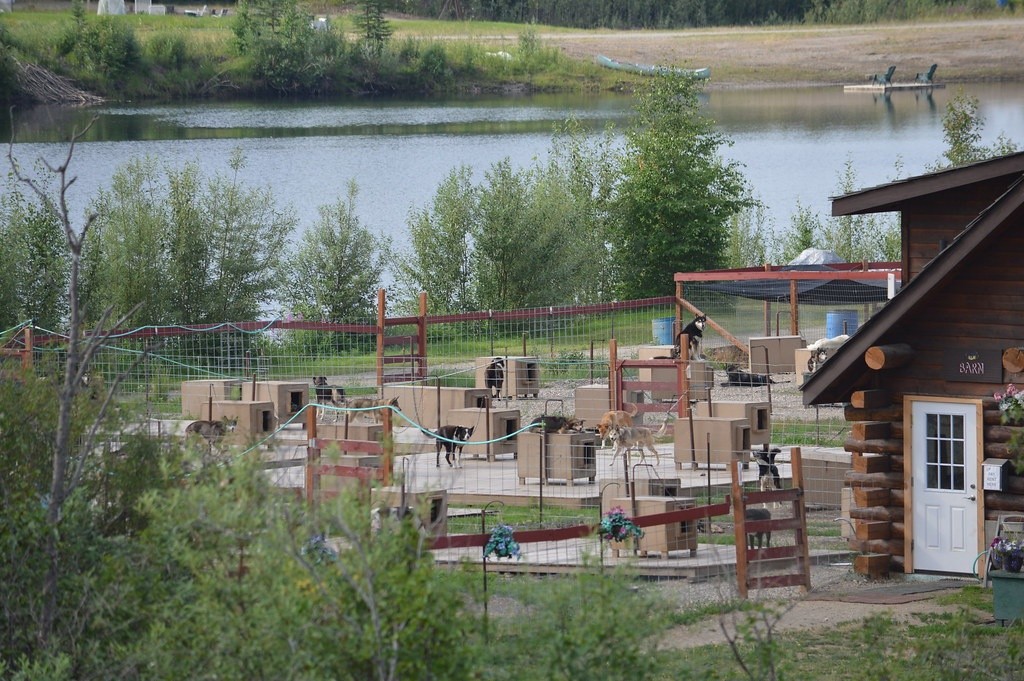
[872,65,896,88]
[914,63,938,83]
[983,514,1024,588]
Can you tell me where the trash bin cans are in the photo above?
[825,309,860,339]
[653,317,683,345]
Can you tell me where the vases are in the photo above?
[1003,555,1022,572]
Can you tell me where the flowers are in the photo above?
[601,505,645,543]
[993,383,1024,424]
[990,534,1024,569]
[483,523,522,561]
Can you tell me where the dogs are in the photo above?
[596,402,638,449]
[745,508,770,550]
[529,416,586,434]
[697,520,724,534]
[720,363,791,387]
[652,313,707,361]
[184,416,239,454]
[808,347,828,373]
[331,387,402,425]
[807,335,850,350]
[421,425,475,469]
[605,419,667,465]
[313,376,357,422]
[752,447,782,508]
[483,357,505,402]
